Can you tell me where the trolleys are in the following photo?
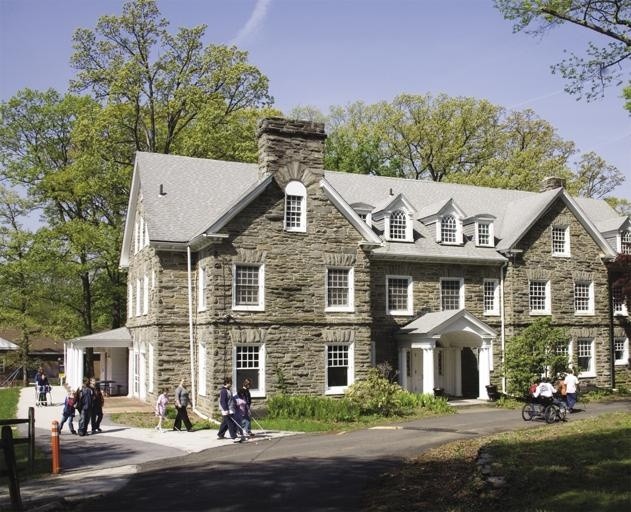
[35,384,54,406]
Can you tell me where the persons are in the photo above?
[89,377,104,433]
[36,366,50,407]
[60,389,79,434]
[565,368,581,413]
[234,388,252,440]
[239,376,256,436]
[39,373,47,406]
[78,378,93,434]
[217,376,242,442]
[173,379,194,432]
[561,377,568,401]
[154,387,169,432]
[532,382,538,393]
[535,378,563,406]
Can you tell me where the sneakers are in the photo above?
[71,428,103,436]
[217,431,255,443]
[189,428,194,432]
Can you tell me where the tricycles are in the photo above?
[517,396,567,423]
